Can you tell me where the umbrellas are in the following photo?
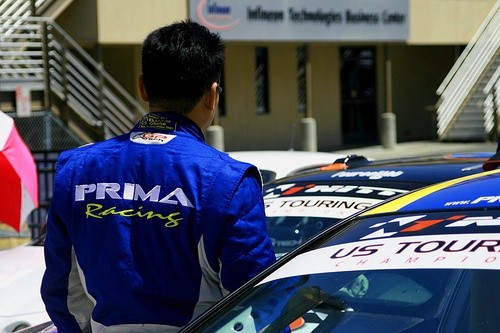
[0,107,38,234]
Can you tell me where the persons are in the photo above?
[41,21,276,333]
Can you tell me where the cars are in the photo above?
[0,151,500,333]
